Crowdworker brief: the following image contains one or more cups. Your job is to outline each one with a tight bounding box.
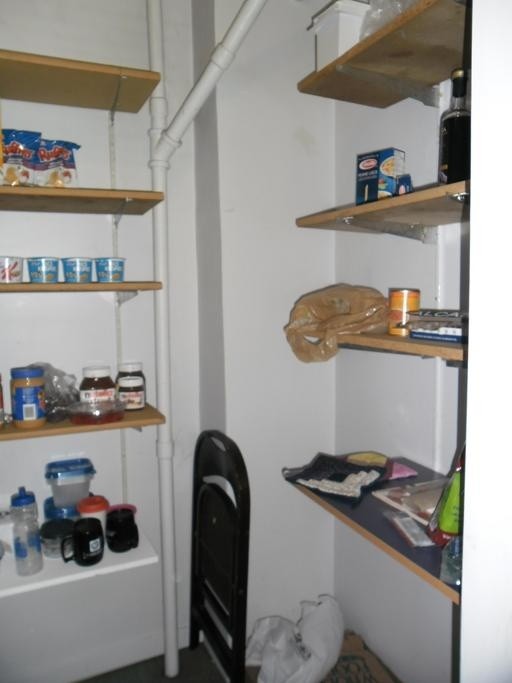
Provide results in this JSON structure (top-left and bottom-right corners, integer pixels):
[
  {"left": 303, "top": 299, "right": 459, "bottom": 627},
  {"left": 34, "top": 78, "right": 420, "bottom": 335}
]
[
  {"left": 61, "top": 517, "right": 104, "bottom": 567},
  {"left": 76, "top": 494, "right": 109, "bottom": 535},
  {"left": 106, "top": 508, "right": 138, "bottom": 553}
]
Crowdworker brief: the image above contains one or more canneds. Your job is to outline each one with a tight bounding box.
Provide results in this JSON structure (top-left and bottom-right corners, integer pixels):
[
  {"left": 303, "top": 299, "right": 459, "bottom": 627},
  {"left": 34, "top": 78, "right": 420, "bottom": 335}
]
[
  {"left": 386, "top": 286, "right": 422, "bottom": 337},
  {"left": 116, "top": 363, "right": 147, "bottom": 413},
  {"left": 10, "top": 366, "right": 48, "bottom": 430},
  {"left": 78, "top": 365, "right": 117, "bottom": 420}
]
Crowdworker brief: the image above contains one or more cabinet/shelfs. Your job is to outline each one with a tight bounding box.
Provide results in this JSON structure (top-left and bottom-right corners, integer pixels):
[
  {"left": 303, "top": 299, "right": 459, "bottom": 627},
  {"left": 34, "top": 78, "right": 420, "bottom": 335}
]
[
  {"left": 282, "top": 0, "right": 462, "bottom": 606},
  {"left": 1, "top": 46, "right": 165, "bottom": 598}
]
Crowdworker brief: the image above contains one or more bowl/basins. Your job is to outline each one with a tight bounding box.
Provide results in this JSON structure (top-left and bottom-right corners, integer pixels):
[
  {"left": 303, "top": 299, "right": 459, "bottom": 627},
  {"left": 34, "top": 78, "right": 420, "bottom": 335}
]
[{"left": 40, "top": 518, "right": 75, "bottom": 560}]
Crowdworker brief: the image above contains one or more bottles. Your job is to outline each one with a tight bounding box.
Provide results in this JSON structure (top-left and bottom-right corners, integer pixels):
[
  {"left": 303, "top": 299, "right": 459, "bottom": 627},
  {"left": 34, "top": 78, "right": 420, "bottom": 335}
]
[
  {"left": 78, "top": 360, "right": 147, "bottom": 413},
  {"left": 439, "top": 65, "right": 471, "bottom": 184},
  {"left": 10, "top": 486, "right": 43, "bottom": 577}
]
